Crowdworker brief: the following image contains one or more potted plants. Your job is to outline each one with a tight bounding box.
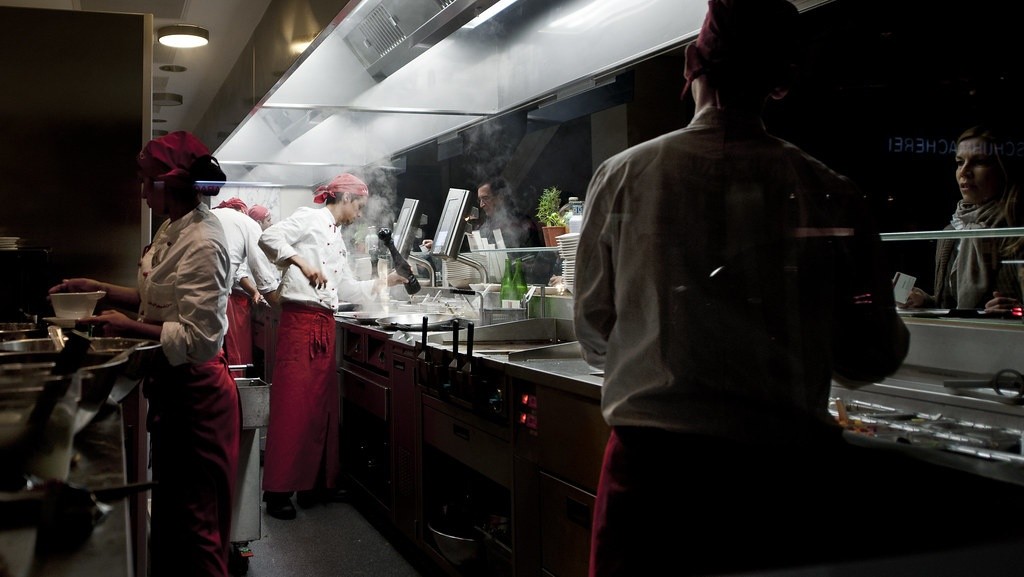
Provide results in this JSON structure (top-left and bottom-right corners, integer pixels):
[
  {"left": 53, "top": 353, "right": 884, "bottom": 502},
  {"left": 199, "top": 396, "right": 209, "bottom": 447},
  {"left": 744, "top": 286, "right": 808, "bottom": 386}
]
[{"left": 535, "top": 186, "right": 567, "bottom": 247}]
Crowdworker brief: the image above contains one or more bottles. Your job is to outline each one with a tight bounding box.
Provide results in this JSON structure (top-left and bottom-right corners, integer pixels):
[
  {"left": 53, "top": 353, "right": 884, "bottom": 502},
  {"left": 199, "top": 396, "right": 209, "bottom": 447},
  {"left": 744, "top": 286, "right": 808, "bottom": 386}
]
[
  {"left": 378, "top": 227, "right": 421, "bottom": 295},
  {"left": 364, "top": 225, "right": 378, "bottom": 252},
  {"left": 559, "top": 196, "right": 583, "bottom": 223},
  {"left": 500, "top": 258, "right": 514, "bottom": 308},
  {"left": 512, "top": 257, "right": 529, "bottom": 309}
]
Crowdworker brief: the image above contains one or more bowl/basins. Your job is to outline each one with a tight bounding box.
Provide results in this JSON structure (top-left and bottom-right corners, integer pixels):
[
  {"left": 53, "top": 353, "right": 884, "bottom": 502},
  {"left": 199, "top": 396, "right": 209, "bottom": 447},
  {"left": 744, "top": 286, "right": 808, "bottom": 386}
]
[
  {"left": 0, "top": 321, "right": 163, "bottom": 490},
  {"left": 336, "top": 303, "right": 457, "bottom": 331},
  {"left": 428, "top": 515, "right": 479, "bottom": 573},
  {"left": 50, "top": 291, "right": 100, "bottom": 318}
]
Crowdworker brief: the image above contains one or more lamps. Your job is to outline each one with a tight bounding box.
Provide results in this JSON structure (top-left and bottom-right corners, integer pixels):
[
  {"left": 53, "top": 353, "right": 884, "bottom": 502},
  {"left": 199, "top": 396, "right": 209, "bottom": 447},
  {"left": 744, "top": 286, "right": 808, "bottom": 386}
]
[
  {"left": 158, "top": 26, "right": 209, "bottom": 48},
  {"left": 153, "top": 93, "right": 182, "bottom": 106}
]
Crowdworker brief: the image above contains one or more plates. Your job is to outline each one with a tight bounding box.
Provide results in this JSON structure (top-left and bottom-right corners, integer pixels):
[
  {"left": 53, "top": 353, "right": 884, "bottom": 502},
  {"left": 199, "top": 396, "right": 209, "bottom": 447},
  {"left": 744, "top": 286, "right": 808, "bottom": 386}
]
[
  {"left": 556, "top": 232, "right": 579, "bottom": 295},
  {"left": 445, "top": 252, "right": 488, "bottom": 288},
  {"left": 44, "top": 317, "right": 94, "bottom": 328},
  {"left": 355, "top": 258, "right": 374, "bottom": 280},
  {"left": 416, "top": 279, "right": 430, "bottom": 285},
  {"left": 0, "top": 237, "right": 21, "bottom": 249}
]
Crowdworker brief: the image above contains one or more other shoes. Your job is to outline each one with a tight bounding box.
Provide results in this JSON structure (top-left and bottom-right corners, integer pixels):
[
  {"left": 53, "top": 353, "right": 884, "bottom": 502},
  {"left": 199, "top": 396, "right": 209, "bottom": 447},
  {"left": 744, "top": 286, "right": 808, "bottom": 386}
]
[
  {"left": 319, "top": 488, "right": 337, "bottom": 496},
  {"left": 267, "top": 495, "right": 296, "bottom": 519}
]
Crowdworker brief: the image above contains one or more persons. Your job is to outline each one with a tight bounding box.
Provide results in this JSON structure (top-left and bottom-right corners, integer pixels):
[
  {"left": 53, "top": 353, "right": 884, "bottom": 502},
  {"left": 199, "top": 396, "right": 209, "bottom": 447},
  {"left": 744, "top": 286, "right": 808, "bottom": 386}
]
[
  {"left": 234, "top": 204, "right": 282, "bottom": 371},
  {"left": 207, "top": 198, "right": 279, "bottom": 380},
  {"left": 573, "top": 1, "right": 909, "bottom": 577},
  {"left": 469, "top": 176, "right": 540, "bottom": 250},
  {"left": 896, "top": 127, "right": 1024, "bottom": 316},
  {"left": 258, "top": 173, "right": 408, "bottom": 521},
  {"left": 48, "top": 129, "right": 244, "bottom": 577}
]
